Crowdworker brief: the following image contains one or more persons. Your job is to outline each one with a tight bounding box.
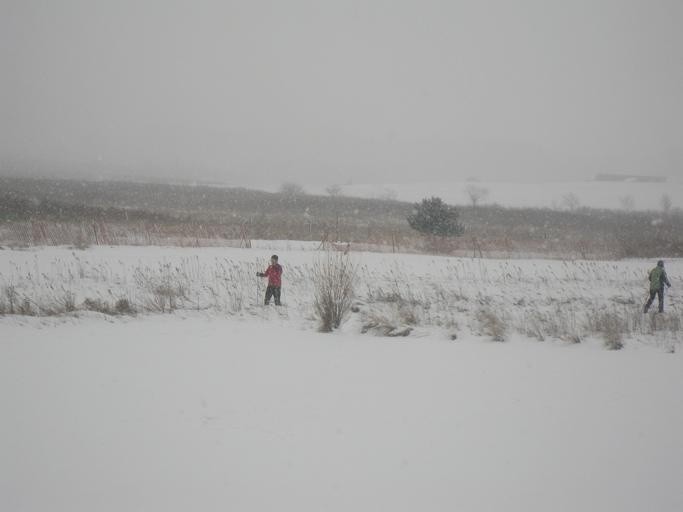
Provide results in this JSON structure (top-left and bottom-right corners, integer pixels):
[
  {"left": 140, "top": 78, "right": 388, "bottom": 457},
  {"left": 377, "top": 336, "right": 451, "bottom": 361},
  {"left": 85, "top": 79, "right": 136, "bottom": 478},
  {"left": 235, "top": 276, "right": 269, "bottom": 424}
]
[
  {"left": 255, "top": 254, "right": 282, "bottom": 305},
  {"left": 643, "top": 260, "right": 671, "bottom": 314}
]
